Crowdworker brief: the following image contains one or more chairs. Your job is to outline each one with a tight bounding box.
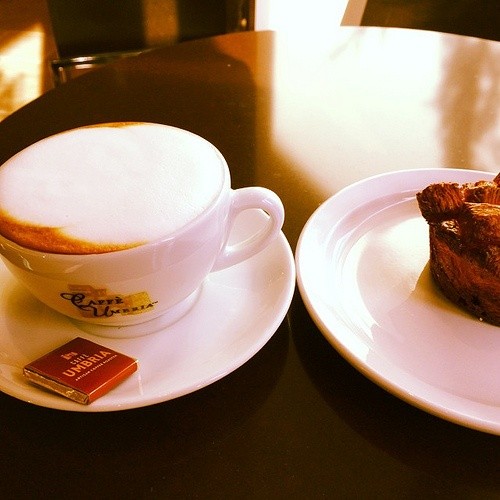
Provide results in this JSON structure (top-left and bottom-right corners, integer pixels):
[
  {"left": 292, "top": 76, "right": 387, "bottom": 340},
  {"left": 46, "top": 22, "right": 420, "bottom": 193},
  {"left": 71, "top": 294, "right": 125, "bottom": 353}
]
[
  {"left": 341, "top": 0, "right": 500, "bottom": 41},
  {"left": 46, "top": 0, "right": 255, "bottom": 88}
]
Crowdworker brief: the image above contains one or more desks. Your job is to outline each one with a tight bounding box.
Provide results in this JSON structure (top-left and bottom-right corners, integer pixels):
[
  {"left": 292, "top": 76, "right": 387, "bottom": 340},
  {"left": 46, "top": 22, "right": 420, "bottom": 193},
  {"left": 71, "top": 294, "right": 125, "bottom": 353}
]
[{"left": 0, "top": 26, "right": 499, "bottom": 500}]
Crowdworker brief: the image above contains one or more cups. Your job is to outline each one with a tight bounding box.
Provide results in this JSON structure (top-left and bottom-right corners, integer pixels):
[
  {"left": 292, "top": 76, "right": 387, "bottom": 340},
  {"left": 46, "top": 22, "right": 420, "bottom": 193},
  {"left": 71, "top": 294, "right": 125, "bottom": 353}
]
[{"left": 0, "top": 119, "right": 285, "bottom": 340}]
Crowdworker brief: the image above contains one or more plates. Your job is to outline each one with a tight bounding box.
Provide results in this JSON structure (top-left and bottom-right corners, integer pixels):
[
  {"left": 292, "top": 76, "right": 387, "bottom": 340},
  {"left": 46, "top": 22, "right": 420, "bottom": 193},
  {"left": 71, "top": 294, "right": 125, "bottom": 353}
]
[
  {"left": 0, "top": 185, "right": 297, "bottom": 414},
  {"left": 295, "top": 166, "right": 500, "bottom": 435}
]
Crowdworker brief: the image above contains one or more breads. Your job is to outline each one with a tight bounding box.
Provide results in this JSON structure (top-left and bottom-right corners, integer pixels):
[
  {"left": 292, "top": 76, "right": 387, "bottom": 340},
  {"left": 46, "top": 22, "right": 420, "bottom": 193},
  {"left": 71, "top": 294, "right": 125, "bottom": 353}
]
[{"left": 416, "top": 172, "right": 500, "bottom": 329}]
